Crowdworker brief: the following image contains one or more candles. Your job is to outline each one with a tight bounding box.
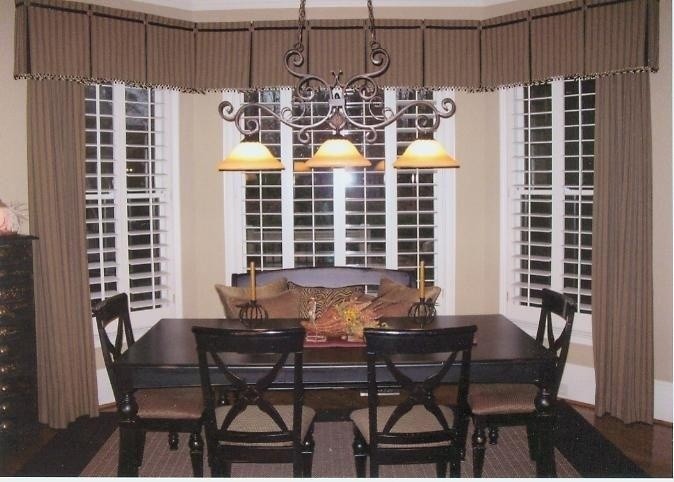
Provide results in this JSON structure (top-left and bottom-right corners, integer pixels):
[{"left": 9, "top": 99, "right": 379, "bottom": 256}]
[
  {"left": 420, "top": 261, "right": 425, "bottom": 298},
  {"left": 250, "top": 261, "right": 255, "bottom": 300}
]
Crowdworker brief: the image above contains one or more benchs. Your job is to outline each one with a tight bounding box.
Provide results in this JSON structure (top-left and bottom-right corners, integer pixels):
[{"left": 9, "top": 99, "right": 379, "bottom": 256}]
[{"left": 231, "top": 267, "right": 414, "bottom": 294}]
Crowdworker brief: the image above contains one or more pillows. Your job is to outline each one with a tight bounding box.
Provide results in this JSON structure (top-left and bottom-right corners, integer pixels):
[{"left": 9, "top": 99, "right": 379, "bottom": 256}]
[
  {"left": 215, "top": 277, "right": 298, "bottom": 319},
  {"left": 372, "top": 277, "right": 441, "bottom": 318},
  {"left": 287, "top": 281, "right": 363, "bottom": 319}
]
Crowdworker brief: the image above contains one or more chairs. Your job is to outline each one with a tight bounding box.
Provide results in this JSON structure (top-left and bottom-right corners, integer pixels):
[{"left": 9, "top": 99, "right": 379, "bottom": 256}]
[
  {"left": 468, "top": 290, "right": 576, "bottom": 476},
  {"left": 345, "top": 323, "right": 478, "bottom": 477},
  {"left": 190, "top": 321, "right": 317, "bottom": 479},
  {"left": 93, "top": 289, "right": 208, "bottom": 477}
]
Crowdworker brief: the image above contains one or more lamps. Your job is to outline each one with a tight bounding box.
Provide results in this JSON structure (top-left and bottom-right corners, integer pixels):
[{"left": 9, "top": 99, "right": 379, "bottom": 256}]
[{"left": 217, "top": 0, "right": 461, "bottom": 171}]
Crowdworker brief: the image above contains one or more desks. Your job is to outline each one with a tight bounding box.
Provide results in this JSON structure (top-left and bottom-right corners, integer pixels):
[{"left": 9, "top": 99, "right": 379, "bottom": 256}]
[{"left": 109, "top": 313, "right": 557, "bottom": 477}]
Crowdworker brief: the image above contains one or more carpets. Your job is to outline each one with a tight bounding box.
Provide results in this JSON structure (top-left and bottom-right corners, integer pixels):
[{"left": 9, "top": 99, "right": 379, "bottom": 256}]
[{"left": 12, "top": 396, "right": 651, "bottom": 478}]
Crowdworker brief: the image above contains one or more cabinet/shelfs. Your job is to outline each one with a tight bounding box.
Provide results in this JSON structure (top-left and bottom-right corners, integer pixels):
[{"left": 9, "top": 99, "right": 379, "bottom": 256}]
[{"left": 0, "top": 235, "right": 41, "bottom": 455}]
